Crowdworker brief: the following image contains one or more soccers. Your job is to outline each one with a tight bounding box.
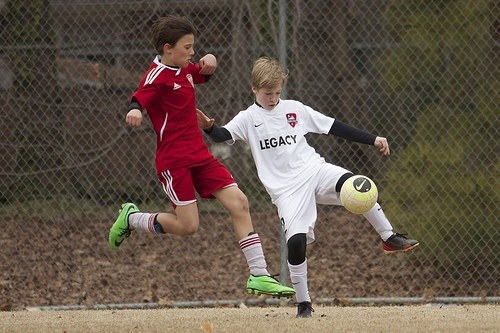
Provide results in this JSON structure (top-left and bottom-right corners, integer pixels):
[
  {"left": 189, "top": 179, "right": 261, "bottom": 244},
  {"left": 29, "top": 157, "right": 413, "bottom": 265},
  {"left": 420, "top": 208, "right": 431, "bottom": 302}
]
[{"left": 340, "top": 175, "right": 378, "bottom": 214}]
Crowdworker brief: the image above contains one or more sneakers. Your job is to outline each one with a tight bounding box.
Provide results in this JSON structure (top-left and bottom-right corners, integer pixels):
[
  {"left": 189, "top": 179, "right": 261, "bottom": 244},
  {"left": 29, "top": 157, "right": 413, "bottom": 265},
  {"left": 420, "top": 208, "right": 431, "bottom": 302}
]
[
  {"left": 294, "top": 301, "right": 314, "bottom": 318},
  {"left": 109, "top": 203, "right": 140, "bottom": 249},
  {"left": 246, "top": 274, "right": 296, "bottom": 299},
  {"left": 379, "top": 232, "right": 419, "bottom": 255}
]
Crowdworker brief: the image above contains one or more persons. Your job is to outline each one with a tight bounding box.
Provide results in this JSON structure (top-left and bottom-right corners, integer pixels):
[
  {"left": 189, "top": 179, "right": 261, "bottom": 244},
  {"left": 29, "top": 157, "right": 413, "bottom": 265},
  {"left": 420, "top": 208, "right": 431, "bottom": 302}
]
[
  {"left": 109, "top": 17, "right": 296, "bottom": 299},
  {"left": 196, "top": 58, "right": 419, "bottom": 318}
]
